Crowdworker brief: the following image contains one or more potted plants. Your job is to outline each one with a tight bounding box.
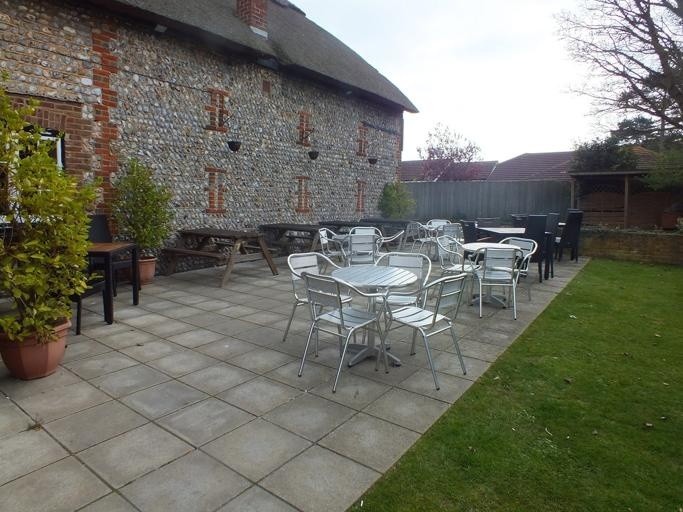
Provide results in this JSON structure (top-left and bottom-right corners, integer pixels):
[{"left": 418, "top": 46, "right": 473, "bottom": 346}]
[
  {"left": 0, "top": 72, "right": 103, "bottom": 380},
  {"left": 106, "top": 157, "right": 175, "bottom": 283}
]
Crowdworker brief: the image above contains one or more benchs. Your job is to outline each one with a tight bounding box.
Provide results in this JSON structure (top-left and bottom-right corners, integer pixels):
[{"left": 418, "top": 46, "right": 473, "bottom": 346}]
[
  {"left": 163, "top": 248, "right": 224, "bottom": 260},
  {"left": 214, "top": 241, "right": 280, "bottom": 256}
]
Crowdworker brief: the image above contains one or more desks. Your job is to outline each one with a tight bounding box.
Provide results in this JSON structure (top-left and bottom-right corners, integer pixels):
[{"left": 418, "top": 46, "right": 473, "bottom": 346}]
[
  {"left": 86, "top": 242, "right": 140, "bottom": 324},
  {"left": 179, "top": 227, "right": 279, "bottom": 289},
  {"left": 259, "top": 222, "right": 337, "bottom": 252}
]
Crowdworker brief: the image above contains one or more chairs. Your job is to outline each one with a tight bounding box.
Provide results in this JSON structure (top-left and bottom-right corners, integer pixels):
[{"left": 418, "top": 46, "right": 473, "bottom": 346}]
[{"left": 73, "top": 279, "right": 109, "bottom": 335}]
